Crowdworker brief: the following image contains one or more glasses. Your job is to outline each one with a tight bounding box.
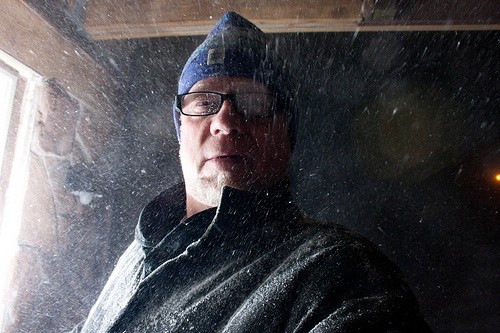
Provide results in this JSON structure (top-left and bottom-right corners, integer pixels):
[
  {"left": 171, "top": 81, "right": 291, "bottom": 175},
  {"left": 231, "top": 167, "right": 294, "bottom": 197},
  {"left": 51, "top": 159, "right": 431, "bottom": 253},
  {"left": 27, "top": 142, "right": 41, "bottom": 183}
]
[{"left": 176, "top": 91, "right": 280, "bottom": 117}]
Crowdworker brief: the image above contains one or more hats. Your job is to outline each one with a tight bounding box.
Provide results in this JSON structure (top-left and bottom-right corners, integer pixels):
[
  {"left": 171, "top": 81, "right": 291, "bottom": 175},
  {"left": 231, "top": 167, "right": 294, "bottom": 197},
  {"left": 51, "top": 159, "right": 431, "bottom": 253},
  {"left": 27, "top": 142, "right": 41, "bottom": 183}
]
[{"left": 172, "top": 10, "right": 286, "bottom": 144}]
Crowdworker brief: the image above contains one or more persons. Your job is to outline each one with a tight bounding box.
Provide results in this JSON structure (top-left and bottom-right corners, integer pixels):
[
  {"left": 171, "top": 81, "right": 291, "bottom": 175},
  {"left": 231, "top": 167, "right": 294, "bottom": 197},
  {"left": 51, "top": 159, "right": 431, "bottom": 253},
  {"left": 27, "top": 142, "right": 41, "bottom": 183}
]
[{"left": 68, "top": 11, "right": 433, "bottom": 333}]
